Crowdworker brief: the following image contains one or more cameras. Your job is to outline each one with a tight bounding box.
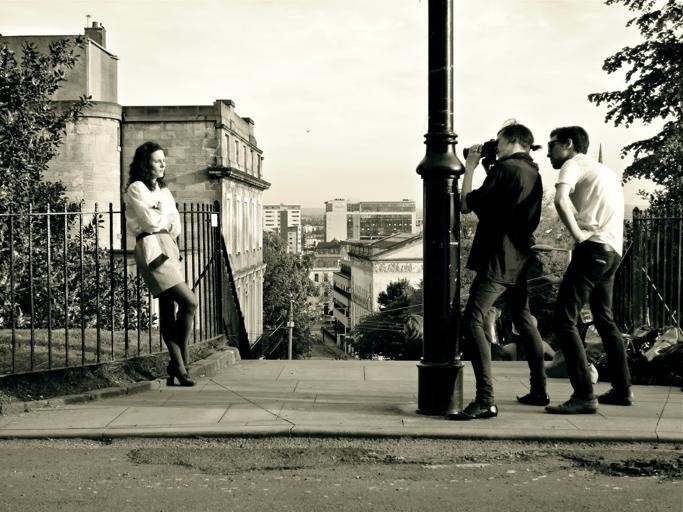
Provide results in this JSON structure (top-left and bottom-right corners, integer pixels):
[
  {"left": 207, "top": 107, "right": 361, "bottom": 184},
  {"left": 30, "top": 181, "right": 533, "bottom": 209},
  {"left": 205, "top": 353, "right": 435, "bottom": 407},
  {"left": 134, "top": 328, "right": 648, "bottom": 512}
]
[{"left": 463, "top": 138, "right": 498, "bottom": 174}]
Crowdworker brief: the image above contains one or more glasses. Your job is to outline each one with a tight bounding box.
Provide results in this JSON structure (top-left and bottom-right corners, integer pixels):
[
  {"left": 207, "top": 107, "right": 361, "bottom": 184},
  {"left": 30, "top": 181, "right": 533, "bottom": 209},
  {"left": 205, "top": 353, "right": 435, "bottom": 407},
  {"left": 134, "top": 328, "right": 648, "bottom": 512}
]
[{"left": 547, "top": 139, "right": 559, "bottom": 148}]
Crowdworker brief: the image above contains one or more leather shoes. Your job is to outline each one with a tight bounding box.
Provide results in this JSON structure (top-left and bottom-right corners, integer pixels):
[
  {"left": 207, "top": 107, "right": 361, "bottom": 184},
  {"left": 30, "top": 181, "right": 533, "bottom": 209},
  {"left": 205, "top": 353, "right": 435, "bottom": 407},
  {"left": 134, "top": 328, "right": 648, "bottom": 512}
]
[
  {"left": 545, "top": 396, "right": 600, "bottom": 413},
  {"left": 597, "top": 388, "right": 632, "bottom": 405},
  {"left": 518, "top": 393, "right": 550, "bottom": 406},
  {"left": 449, "top": 402, "right": 498, "bottom": 419}
]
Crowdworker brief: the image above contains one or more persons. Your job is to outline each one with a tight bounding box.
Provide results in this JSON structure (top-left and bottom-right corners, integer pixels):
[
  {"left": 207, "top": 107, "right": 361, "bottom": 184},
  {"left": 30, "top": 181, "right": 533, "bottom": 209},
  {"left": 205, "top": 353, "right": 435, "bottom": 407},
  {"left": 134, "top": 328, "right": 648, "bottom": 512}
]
[
  {"left": 127, "top": 141, "right": 198, "bottom": 386},
  {"left": 446, "top": 122, "right": 551, "bottom": 421},
  {"left": 544, "top": 127, "right": 632, "bottom": 413}
]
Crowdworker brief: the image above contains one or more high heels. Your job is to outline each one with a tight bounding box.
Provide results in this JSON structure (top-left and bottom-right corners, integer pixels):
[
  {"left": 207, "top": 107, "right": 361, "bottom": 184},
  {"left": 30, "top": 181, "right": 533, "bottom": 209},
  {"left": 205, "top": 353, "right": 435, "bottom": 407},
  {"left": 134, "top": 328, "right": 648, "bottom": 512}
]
[{"left": 167, "top": 360, "right": 196, "bottom": 386}]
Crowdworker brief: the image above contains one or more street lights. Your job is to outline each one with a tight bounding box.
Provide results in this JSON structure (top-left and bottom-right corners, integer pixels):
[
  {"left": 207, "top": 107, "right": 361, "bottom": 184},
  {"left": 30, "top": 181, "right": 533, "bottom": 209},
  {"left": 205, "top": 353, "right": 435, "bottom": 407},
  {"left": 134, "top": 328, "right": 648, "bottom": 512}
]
[
  {"left": 336, "top": 307, "right": 347, "bottom": 356},
  {"left": 287, "top": 299, "right": 296, "bottom": 360}
]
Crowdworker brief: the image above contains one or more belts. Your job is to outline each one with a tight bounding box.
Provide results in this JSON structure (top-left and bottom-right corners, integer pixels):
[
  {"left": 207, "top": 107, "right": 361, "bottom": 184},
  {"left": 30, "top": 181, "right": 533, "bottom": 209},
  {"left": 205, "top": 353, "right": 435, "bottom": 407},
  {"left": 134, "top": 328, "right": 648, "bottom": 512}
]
[{"left": 581, "top": 242, "right": 614, "bottom": 252}]
